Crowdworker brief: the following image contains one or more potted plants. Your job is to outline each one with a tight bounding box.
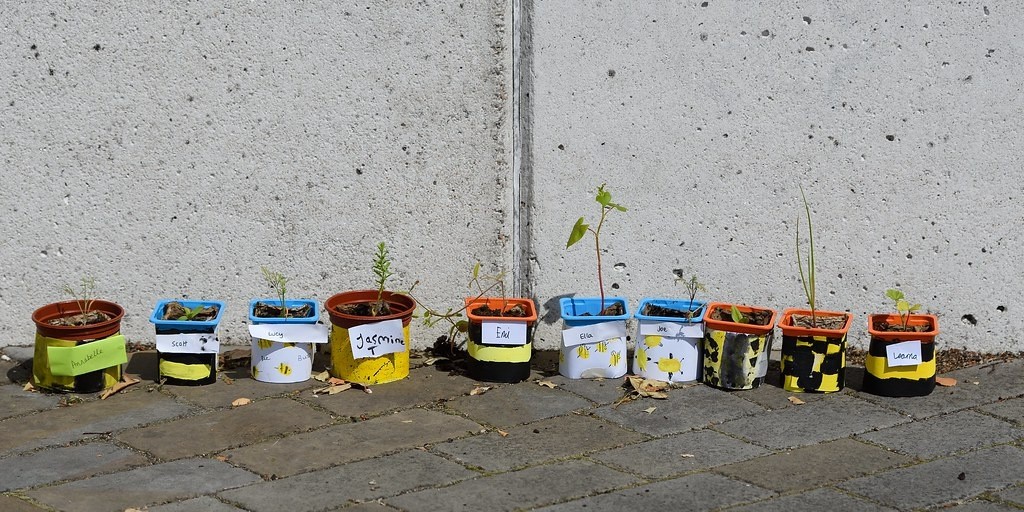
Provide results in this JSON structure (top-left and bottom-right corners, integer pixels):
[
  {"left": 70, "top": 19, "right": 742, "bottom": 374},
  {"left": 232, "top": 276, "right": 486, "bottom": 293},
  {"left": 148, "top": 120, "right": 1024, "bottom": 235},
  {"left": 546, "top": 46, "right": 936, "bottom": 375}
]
[
  {"left": 247, "top": 266, "right": 329, "bottom": 384},
  {"left": 861, "top": 289, "right": 938, "bottom": 399},
  {"left": 632, "top": 267, "right": 708, "bottom": 382},
  {"left": 703, "top": 304, "right": 778, "bottom": 391},
  {"left": 390, "top": 260, "right": 538, "bottom": 383},
  {"left": 558, "top": 183, "right": 631, "bottom": 379},
  {"left": 33, "top": 277, "right": 128, "bottom": 393},
  {"left": 324, "top": 242, "right": 416, "bottom": 387},
  {"left": 776, "top": 183, "right": 854, "bottom": 393},
  {"left": 149, "top": 300, "right": 224, "bottom": 386}
]
